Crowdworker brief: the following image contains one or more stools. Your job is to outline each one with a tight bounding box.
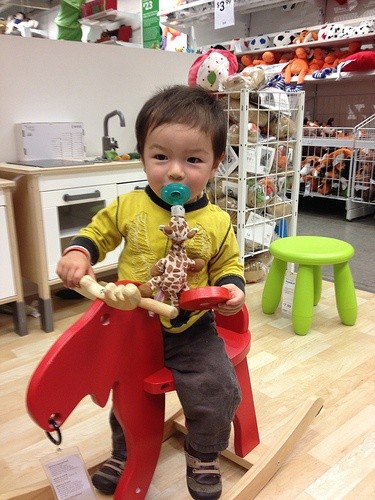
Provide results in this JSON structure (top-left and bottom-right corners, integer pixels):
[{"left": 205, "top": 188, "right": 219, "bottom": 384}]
[{"left": 261, "top": 235, "right": 357, "bottom": 336}]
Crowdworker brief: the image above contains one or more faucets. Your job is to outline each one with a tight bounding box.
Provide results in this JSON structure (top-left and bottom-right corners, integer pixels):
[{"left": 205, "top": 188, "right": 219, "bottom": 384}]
[{"left": 101, "top": 109, "right": 126, "bottom": 159}]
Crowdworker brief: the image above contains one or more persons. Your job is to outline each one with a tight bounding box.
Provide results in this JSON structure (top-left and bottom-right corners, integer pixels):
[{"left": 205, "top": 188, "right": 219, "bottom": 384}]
[{"left": 55, "top": 84, "right": 247, "bottom": 500}]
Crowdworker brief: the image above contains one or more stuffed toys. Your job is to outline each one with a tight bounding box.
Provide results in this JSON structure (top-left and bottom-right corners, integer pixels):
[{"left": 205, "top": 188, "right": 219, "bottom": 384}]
[
  {"left": 301, "top": 118, "right": 375, "bottom": 201},
  {"left": 187, "top": 48, "right": 291, "bottom": 283},
  {"left": 139, "top": 205, "right": 205, "bottom": 312},
  {"left": 241, "top": 40, "right": 375, "bottom": 93}
]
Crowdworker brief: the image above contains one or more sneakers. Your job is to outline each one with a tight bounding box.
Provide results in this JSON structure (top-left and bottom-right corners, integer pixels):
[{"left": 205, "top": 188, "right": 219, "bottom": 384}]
[
  {"left": 91, "top": 454, "right": 126, "bottom": 490},
  {"left": 184, "top": 436, "right": 222, "bottom": 500}
]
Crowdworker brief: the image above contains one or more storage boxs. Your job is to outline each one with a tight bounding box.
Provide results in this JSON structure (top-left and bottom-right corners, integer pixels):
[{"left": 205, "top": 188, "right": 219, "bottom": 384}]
[{"left": 14, "top": 121, "right": 87, "bottom": 163}]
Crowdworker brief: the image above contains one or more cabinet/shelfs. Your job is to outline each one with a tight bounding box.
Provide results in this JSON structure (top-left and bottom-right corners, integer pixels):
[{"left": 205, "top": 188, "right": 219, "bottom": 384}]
[
  {"left": 0, "top": 169, "right": 151, "bottom": 334},
  {"left": 77, "top": 8, "right": 143, "bottom": 49},
  {"left": 156, "top": 0, "right": 375, "bottom": 150}
]
